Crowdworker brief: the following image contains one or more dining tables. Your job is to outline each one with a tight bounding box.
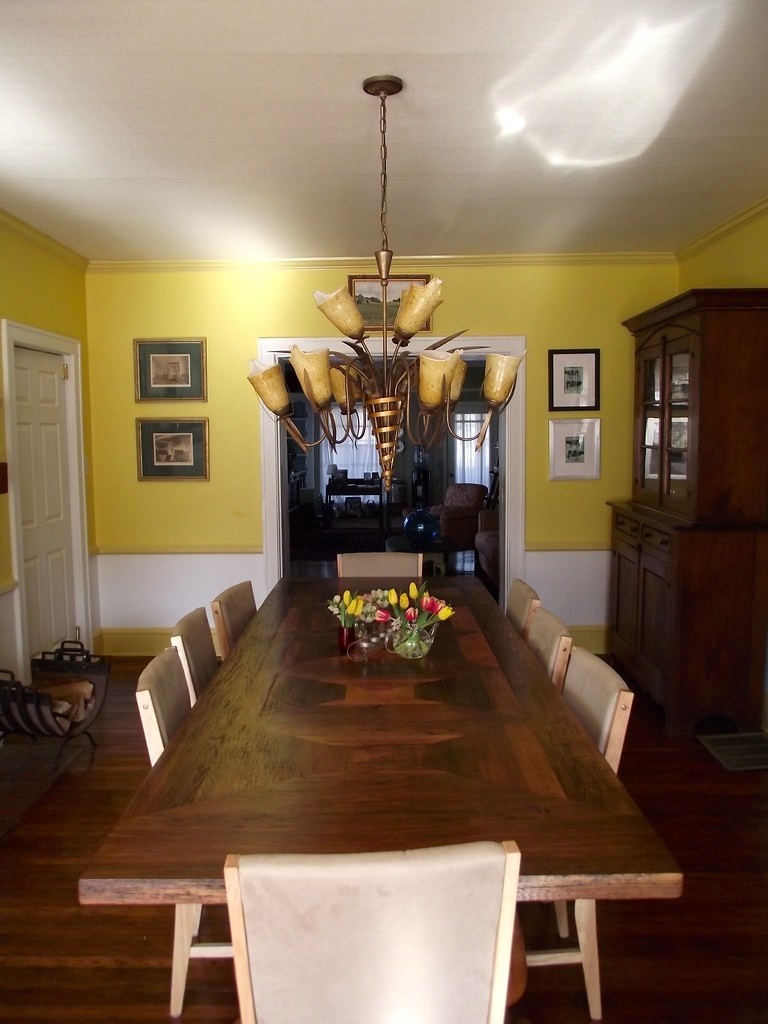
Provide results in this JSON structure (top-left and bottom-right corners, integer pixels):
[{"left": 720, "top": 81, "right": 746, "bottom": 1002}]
[{"left": 73, "top": 572, "right": 685, "bottom": 1024}]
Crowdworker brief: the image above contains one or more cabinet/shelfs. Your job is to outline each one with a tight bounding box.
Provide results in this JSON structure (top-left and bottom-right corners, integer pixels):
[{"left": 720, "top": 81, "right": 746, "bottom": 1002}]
[
  {"left": 603, "top": 501, "right": 687, "bottom": 734},
  {"left": 619, "top": 292, "right": 703, "bottom": 529}
]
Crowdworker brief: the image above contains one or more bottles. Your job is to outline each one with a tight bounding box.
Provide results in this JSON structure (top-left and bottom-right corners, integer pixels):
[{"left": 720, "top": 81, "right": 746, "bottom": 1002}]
[{"left": 413, "top": 444, "right": 429, "bottom": 467}]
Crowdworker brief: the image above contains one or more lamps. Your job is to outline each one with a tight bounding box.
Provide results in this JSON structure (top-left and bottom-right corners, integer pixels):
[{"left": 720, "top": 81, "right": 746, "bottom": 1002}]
[
  {"left": 327, "top": 464, "right": 339, "bottom": 489},
  {"left": 135, "top": 416, "right": 212, "bottom": 484},
  {"left": 248, "top": 71, "right": 527, "bottom": 493},
  {"left": 548, "top": 418, "right": 601, "bottom": 482},
  {"left": 547, "top": 347, "right": 601, "bottom": 413},
  {"left": 132, "top": 336, "right": 210, "bottom": 405}
]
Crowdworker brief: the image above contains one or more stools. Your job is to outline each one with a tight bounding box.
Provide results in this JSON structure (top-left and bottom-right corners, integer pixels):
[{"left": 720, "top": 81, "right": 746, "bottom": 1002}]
[{"left": 1, "top": 639, "right": 112, "bottom": 775}]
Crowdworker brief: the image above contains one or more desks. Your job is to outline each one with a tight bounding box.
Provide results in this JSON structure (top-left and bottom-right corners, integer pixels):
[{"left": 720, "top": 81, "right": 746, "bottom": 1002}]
[{"left": 324, "top": 478, "right": 384, "bottom": 534}]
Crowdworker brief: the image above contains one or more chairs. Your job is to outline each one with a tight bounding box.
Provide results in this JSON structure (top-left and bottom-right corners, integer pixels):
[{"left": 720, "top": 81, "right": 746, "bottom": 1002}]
[
  {"left": 524, "top": 603, "right": 573, "bottom": 700},
  {"left": 134, "top": 646, "right": 193, "bottom": 770},
  {"left": 508, "top": 576, "right": 541, "bottom": 641},
  {"left": 211, "top": 580, "right": 261, "bottom": 664},
  {"left": 475, "top": 509, "right": 501, "bottom": 588},
  {"left": 522, "top": 646, "right": 636, "bottom": 1021},
  {"left": 168, "top": 608, "right": 220, "bottom": 709},
  {"left": 220, "top": 839, "right": 524, "bottom": 1024},
  {"left": 401, "top": 482, "right": 488, "bottom": 553},
  {"left": 336, "top": 552, "right": 423, "bottom": 578}
]
